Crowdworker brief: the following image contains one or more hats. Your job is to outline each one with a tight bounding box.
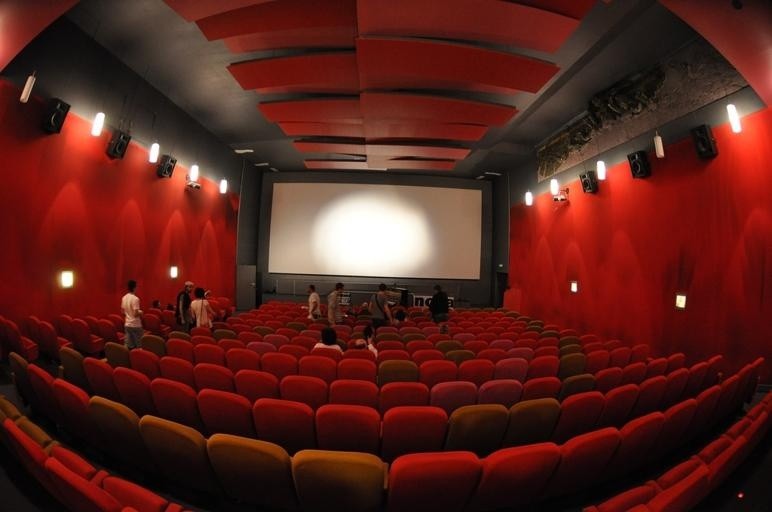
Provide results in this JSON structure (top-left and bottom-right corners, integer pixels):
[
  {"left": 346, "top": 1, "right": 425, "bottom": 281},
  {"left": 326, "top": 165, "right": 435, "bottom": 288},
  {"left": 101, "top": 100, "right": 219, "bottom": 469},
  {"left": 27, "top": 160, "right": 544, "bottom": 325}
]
[{"left": 185, "top": 281, "right": 194, "bottom": 285}]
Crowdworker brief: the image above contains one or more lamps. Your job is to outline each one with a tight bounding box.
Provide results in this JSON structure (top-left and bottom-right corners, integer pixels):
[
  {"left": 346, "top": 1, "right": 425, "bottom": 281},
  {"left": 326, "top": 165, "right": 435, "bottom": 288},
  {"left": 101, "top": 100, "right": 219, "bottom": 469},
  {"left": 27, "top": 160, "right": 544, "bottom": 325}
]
[
  {"left": 721, "top": 86, "right": 741, "bottom": 132},
  {"left": 596, "top": 133, "right": 605, "bottom": 180},
  {"left": 219, "top": 174, "right": 228, "bottom": 194},
  {"left": 148, "top": 138, "right": 159, "bottom": 163},
  {"left": 189, "top": 164, "right": 199, "bottom": 182},
  {"left": 550, "top": 161, "right": 559, "bottom": 197},
  {"left": 525, "top": 185, "right": 533, "bottom": 207},
  {"left": 92, "top": 108, "right": 105, "bottom": 136},
  {"left": 651, "top": 108, "right": 665, "bottom": 158},
  {"left": 19, "top": 70, "right": 37, "bottom": 103}
]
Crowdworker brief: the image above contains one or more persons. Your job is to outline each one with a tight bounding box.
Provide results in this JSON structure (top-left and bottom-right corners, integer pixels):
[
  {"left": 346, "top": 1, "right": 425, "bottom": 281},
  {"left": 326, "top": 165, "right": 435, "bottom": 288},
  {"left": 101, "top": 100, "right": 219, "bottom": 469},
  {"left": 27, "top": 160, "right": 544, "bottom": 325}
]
[
  {"left": 166, "top": 303, "right": 174, "bottom": 311},
  {"left": 429, "top": 284, "right": 449, "bottom": 323},
  {"left": 306, "top": 284, "right": 322, "bottom": 320},
  {"left": 312, "top": 327, "right": 344, "bottom": 354},
  {"left": 350, "top": 333, "right": 378, "bottom": 358},
  {"left": 359, "top": 302, "right": 371, "bottom": 315},
  {"left": 327, "top": 283, "right": 345, "bottom": 325},
  {"left": 368, "top": 284, "right": 392, "bottom": 328},
  {"left": 152, "top": 300, "right": 161, "bottom": 308},
  {"left": 176, "top": 281, "right": 194, "bottom": 332},
  {"left": 121, "top": 280, "right": 144, "bottom": 350},
  {"left": 189, "top": 288, "right": 216, "bottom": 329},
  {"left": 393, "top": 310, "right": 408, "bottom": 322}
]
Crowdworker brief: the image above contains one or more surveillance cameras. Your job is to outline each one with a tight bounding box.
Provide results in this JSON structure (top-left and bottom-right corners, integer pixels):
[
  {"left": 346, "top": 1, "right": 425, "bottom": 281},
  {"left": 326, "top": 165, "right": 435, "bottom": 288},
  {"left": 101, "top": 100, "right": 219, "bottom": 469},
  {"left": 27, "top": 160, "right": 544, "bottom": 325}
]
[
  {"left": 186, "top": 182, "right": 193, "bottom": 188},
  {"left": 553, "top": 196, "right": 558, "bottom": 201},
  {"left": 193, "top": 183, "right": 201, "bottom": 190},
  {"left": 559, "top": 196, "right": 565, "bottom": 201}
]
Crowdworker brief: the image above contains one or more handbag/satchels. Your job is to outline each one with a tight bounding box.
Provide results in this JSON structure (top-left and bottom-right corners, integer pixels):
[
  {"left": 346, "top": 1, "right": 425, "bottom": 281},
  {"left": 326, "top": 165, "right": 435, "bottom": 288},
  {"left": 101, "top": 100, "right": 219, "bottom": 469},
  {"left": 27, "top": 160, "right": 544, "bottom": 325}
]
[
  {"left": 384, "top": 310, "right": 394, "bottom": 320},
  {"left": 201, "top": 323, "right": 209, "bottom": 328}
]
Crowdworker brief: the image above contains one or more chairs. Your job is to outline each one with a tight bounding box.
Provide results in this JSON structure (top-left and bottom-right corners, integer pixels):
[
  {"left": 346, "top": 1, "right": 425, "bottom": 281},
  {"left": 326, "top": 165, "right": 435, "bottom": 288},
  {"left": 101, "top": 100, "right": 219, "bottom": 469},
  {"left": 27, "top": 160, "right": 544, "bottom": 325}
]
[{"left": 0, "top": 297, "right": 772, "bottom": 512}]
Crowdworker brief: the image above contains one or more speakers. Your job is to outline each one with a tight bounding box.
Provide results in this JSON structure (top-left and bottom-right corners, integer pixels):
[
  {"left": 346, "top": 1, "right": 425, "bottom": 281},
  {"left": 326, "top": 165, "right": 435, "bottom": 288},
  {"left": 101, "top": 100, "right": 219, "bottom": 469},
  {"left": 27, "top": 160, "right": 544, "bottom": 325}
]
[
  {"left": 40, "top": 98, "right": 71, "bottom": 134},
  {"left": 579, "top": 171, "right": 597, "bottom": 193},
  {"left": 627, "top": 150, "right": 650, "bottom": 177},
  {"left": 157, "top": 154, "right": 177, "bottom": 179},
  {"left": 106, "top": 130, "right": 132, "bottom": 160},
  {"left": 690, "top": 124, "right": 718, "bottom": 157}
]
